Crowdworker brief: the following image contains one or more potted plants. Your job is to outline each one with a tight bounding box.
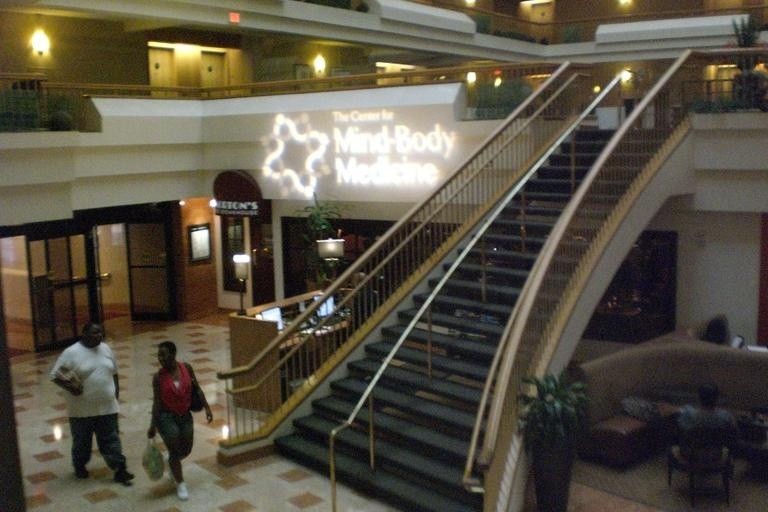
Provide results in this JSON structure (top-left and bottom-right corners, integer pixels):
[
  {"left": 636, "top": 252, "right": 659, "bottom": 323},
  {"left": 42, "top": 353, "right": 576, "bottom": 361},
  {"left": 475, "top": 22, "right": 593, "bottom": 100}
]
[
  {"left": 721, "top": 11, "right": 767, "bottom": 103},
  {"left": 520, "top": 368, "right": 590, "bottom": 511}
]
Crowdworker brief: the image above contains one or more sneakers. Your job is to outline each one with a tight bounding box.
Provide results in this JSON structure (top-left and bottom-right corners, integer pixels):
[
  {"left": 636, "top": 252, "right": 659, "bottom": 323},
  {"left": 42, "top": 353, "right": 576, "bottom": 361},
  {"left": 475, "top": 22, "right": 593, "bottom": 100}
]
[
  {"left": 73, "top": 465, "right": 90, "bottom": 479},
  {"left": 113, "top": 468, "right": 136, "bottom": 482},
  {"left": 164, "top": 453, "right": 175, "bottom": 481},
  {"left": 175, "top": 480, "right": 190, "bottom": 502}
]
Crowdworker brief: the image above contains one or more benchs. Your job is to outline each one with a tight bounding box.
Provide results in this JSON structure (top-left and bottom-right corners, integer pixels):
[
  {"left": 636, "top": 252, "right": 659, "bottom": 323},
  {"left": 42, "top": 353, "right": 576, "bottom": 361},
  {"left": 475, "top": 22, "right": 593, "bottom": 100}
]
[{"left": 576, "top": 339, "right": 768, "bottom": 470}]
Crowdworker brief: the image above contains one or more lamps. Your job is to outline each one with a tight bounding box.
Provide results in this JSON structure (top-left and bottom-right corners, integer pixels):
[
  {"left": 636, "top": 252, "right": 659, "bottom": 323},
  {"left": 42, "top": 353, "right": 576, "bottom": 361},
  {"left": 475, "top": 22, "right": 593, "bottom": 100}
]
[
  {"left": 232, "top": 254, "right": 250, "bottom": 315},
  {"left": 313, "top": 236, "right": 346, "bottom": 266}
]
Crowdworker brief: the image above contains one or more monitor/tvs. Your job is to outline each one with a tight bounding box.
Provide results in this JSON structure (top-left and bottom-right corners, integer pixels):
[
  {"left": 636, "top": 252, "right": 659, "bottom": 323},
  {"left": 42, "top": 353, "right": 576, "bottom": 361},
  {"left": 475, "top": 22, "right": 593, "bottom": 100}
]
[
  {"left": 313, "top": 294, "right": 335, "bottom": 317},
  {"left": 731, "top": 335, "right": 745, "bottom": 349},
  {"left": 261, "top": 306, "right": 283, "bottom": 331}
]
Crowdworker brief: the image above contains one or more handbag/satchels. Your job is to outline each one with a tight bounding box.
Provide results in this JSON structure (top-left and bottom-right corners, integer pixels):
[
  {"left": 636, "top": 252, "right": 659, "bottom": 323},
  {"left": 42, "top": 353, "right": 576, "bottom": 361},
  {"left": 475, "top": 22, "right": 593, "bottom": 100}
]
[{"left": 183, "top": 361, "right": 205, "bottom": 413}]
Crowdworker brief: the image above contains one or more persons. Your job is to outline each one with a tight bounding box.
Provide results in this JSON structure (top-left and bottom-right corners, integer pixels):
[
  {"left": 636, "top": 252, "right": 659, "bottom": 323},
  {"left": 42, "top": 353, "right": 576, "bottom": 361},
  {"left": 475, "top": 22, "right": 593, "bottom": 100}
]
[
  {"left": 49, "top": 322, "right": 136, "bottom": 483},
  {"left": 703, "top": 315, "right": 732, "bottom": 346},
  {"left": 145, "top": 340, "right": 214, "bottom": 503},
  {"left": 670, "top": 380, "right": 742, "bottom": 450}
]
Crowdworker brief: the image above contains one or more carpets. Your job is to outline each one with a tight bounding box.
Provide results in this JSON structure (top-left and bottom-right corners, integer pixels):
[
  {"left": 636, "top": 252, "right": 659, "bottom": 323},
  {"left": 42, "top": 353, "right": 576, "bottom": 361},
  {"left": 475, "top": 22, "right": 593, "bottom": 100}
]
[
  {"left": 66, "top": 306, "right": 130, "bottom": 324},
  {"left": 562, "top": 444, "right": 767, "bottom": 511},
  {"left": 8, "top": 344, "right": 30, "bottom": 359}
]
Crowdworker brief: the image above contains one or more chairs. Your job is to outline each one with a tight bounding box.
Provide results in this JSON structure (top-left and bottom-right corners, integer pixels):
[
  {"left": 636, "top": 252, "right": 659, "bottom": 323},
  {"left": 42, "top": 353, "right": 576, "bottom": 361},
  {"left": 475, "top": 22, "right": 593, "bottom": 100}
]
[{"left": 667, "top": 431, "right": 735, "bottom": 508}]
